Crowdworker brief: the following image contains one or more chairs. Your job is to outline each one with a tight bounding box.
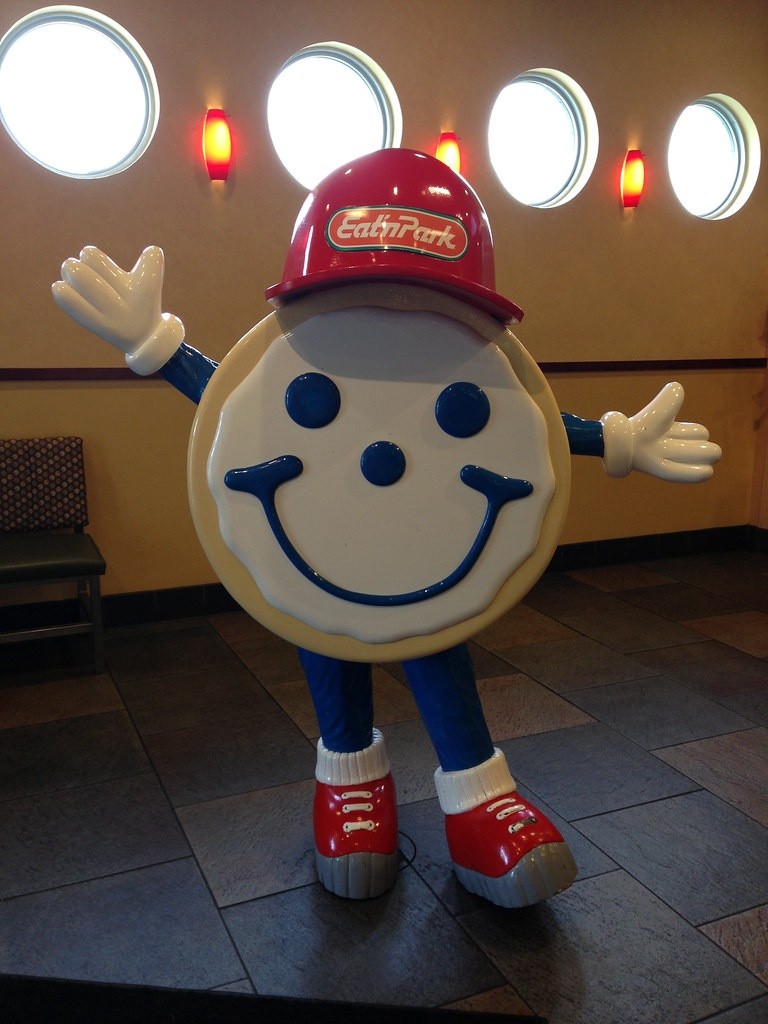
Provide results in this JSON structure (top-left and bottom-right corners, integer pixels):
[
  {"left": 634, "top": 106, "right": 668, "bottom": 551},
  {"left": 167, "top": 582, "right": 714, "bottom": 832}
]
[{"left": 0, "top": 434, "right": 105, "bottom": 674}]
